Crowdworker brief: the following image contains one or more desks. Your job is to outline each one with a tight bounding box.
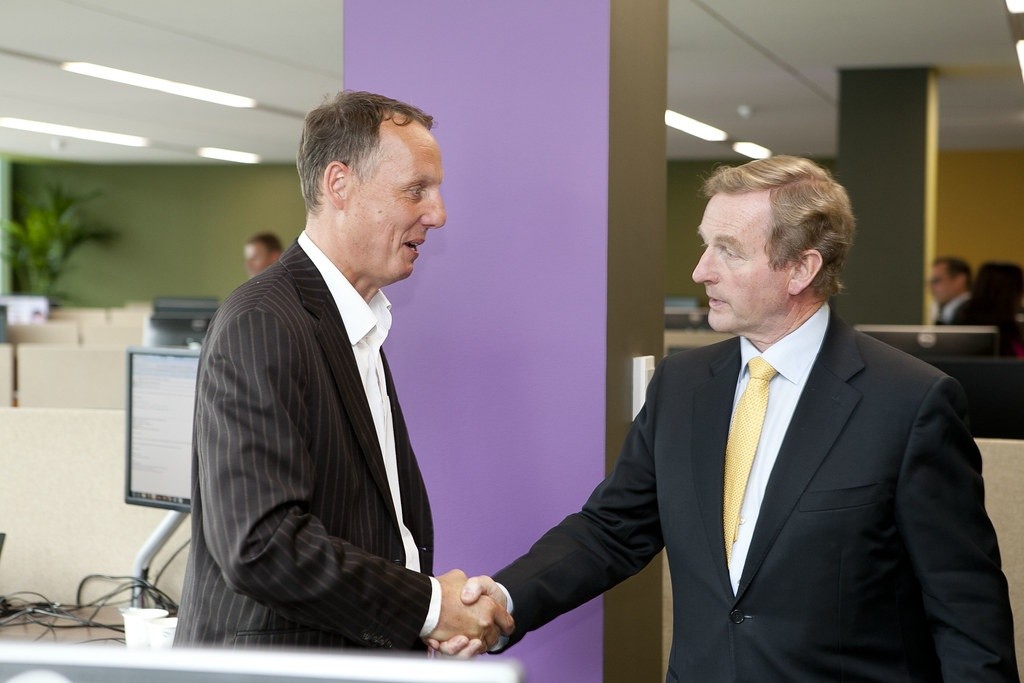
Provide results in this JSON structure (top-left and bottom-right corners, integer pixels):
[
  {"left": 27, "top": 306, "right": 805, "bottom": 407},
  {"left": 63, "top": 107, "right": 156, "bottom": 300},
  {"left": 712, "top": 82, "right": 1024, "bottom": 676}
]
[{"left": 0, "top": 605, "right": 125, "bottom": 647}]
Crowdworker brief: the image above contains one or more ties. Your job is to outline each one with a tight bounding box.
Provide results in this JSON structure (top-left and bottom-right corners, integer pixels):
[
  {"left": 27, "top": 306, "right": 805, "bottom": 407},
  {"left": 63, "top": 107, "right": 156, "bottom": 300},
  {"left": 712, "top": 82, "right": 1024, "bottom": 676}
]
[
  {"left": 930, "top": 274, "right": 952, "bottom": 286},
  {"left": 723, "top": 356, "right": 777, "bottom": 561}
]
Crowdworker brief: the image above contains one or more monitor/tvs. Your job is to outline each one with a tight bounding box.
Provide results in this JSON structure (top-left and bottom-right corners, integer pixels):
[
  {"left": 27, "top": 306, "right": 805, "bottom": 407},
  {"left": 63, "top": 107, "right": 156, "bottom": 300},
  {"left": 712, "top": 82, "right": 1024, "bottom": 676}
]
[
  {"left": 661, "top": 295, "right": 716, "bottom": 331},
  {"left": 851, "top": 325, "right": 1024, "bottom": 439},
  {"left": 123, "top": 295, "right": 223, "bottom": 607},
  {"left": 0, "top": 292, "right": 52, "bottom": 345}
]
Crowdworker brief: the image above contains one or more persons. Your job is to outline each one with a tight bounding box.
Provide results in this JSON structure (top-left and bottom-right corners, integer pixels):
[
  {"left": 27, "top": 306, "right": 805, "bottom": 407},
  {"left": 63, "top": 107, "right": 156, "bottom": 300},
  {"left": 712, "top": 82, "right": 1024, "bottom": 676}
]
[
  {"left": 930, "top": 254, "right": 975, "bottom": 325},
  {"left": 243, "top": 233, "right": 282, "bottom": 279},
  {"left": 949, "top": 261, "right": 1024, "bottom": 358},
  {"left": 170, "top": 91, "right": 515, "bottom": 659},
  {"left": 421, "top": 154, "right": 1021, "bottom": 683}
]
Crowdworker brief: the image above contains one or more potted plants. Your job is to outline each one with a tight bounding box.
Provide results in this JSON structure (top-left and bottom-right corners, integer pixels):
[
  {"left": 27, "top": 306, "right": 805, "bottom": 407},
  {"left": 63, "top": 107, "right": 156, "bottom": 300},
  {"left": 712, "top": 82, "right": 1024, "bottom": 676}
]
[{"left": 0, "top": 177, "right": 123, "bottom": 309}]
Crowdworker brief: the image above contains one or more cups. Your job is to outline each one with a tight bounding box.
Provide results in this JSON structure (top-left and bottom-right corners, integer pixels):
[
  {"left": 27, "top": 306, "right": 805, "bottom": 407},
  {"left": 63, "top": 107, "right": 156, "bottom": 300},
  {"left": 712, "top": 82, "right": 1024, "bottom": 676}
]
[
  {"left": 119, "top": 608, "right": 169, "bottom": 647},
  {"left": 146, "top": 617, "right": 178, "bottom": 648}
]
